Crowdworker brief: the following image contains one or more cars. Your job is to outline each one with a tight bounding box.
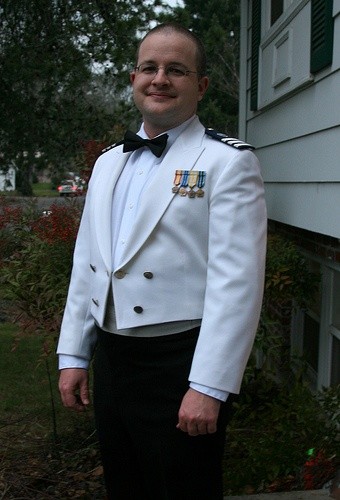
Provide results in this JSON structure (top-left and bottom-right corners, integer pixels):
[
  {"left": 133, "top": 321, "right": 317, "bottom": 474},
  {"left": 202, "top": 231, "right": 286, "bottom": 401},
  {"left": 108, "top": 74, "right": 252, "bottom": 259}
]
[{"left": 58, "top": 180, "right": 84, "bottom": 197}]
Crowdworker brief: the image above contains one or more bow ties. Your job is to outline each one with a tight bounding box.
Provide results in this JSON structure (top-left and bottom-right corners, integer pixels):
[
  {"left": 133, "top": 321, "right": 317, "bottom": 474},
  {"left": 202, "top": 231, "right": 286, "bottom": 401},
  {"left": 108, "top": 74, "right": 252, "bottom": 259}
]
[{"left": 122, "top": 130, "right": 169, "bottom": 157}]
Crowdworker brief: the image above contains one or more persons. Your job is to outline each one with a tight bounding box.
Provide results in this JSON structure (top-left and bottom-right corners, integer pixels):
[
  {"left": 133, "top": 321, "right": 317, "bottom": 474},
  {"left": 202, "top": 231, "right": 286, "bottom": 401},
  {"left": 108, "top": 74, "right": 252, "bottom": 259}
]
[{"left": 54, "top": 20, "right": 270, "bottom": 500}]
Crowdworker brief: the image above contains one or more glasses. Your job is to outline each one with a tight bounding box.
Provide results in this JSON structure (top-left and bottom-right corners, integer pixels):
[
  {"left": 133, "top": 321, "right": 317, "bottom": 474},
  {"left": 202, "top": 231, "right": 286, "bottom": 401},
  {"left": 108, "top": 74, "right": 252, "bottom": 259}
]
[{"left": 133, "top": 63, "right": 204, "bottom": 80}]
